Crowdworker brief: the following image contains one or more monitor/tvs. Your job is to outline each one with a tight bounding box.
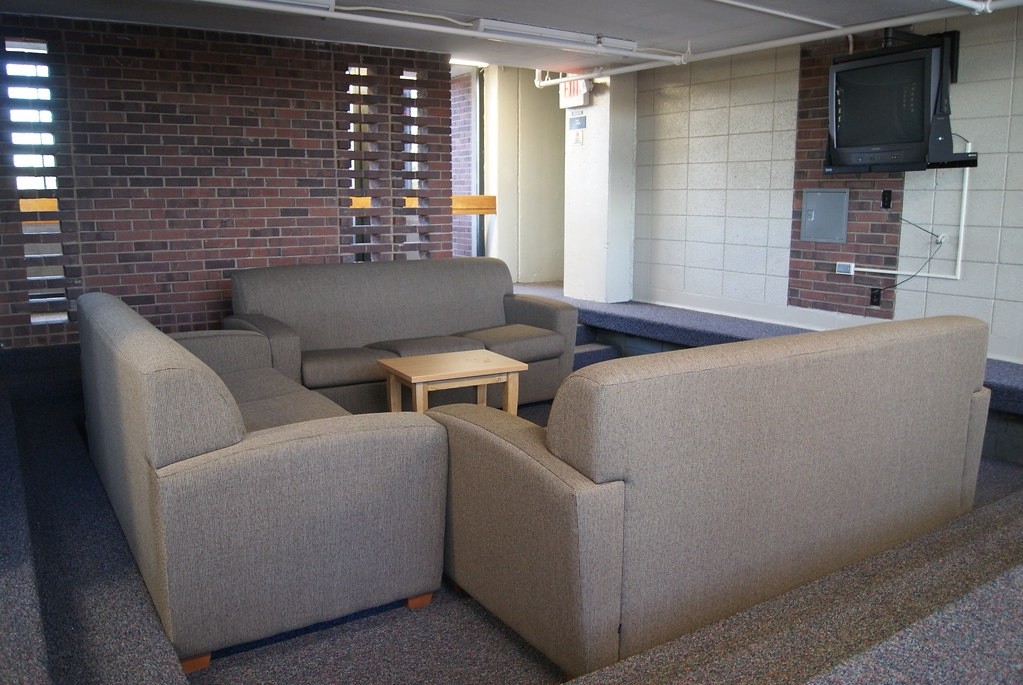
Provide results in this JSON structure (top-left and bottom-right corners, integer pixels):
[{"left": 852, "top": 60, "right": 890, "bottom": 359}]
[{"left": 827, "top": 48, "right": 941, "bottom": 165}]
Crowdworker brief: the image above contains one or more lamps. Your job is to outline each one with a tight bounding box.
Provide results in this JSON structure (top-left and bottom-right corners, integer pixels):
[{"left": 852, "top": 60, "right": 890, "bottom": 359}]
[
  {"left": 220, "top": 0, "right": 335, "bottom": 22},
  {"left": 467, "top": 19, "right": 637, "bottom": 59}
]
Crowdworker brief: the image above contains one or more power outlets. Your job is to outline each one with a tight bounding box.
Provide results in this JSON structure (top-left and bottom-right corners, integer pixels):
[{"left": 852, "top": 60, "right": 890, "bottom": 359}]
[{"left": 870, "top": 288, "right": 881, "bottom": 306}]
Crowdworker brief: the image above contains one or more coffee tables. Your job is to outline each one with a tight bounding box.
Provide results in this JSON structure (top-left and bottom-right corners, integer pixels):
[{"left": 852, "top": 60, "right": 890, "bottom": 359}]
[{"left": 376, "top": 349, "right": 529, "bottom": 416}]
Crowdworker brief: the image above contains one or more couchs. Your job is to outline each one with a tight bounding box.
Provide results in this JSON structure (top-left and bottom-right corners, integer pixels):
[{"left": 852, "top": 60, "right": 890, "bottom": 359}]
[
  {"left": 77, "top": 292, "right": 449, "bottom": 674},
  {"left": 222, "top": 255, "right": 579, "bottom": 416},
  {"left": 422, "top": 314, "right": 991, "bottom": 677}
]
